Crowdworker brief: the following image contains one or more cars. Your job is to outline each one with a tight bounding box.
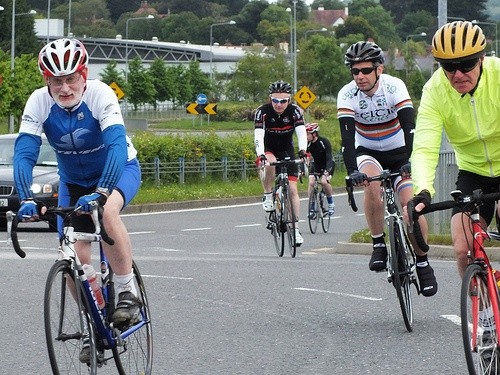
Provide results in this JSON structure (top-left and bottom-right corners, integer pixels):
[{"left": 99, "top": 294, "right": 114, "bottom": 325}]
[{"left": 0, "top": 132, "right": 61, "bottom": 231}]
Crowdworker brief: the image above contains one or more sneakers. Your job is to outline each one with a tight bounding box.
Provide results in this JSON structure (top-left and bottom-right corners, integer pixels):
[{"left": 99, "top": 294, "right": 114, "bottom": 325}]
[
  {"left": 369, "top": 243, "right": 388, "bottom": 271},
  {"left": 328, "top": 201, "right": 335, "bottom": 215},
  {"left": 79, "top": 331, "right": 102, "bottom": 362},
  {"left": 416, "top": 261, "right": 437, "bottom": 297},
  {"left": 307, "top": 209, "right": 314, "bottom": 218},
  {"left": 289, "top": 228, "right": 304, "bottom": 247},
  {"left": 112, "top": 291, "right": 143, "bottom": 327},
  {"left": 262, "top": 192, "right": 274, "bottom": 212}
]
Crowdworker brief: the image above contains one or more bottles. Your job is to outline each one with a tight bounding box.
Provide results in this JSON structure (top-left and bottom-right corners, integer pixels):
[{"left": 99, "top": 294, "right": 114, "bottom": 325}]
[
  {"left": 492, "top": 270, "right": 500, "bottom": 295},
  {"left": 81, "top": 264, "right": 106, "bottom": 311}
]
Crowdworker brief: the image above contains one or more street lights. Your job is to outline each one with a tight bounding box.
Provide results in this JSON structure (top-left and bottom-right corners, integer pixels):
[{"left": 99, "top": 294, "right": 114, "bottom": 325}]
[
  {"left": 210, "top": 21, "right": 236, "bottom": 96},
  {"left": 286, "top": 8, "right": 294, "bottom": 65},
  {"left": 472, "top": 20, "right": 498, "bottom": 57},
  {"left": 406, "top": 32, "right": 427, "bottom": 56},
  {"left": 8, "top": 10, "right": 36, "bottom": 132},
  {"left": 125, "top": 15, "right": 155, "bottom": 117},
  {"left": 305, "top": 28, "right": 327, "bottom": 42}
]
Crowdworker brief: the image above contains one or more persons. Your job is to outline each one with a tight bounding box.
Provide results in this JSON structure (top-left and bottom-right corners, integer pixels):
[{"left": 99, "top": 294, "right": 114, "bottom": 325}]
[
  {"left": 12, "top": 38, "right": 141, "bottom": 364},
  {"left": 337, "top": 40, "right": 439, "bottom": 297},
  {"left": 403, "top": 21, "right": 499, "bottom": 352},
  {"left": 254, "top": 79, "right": 335, "bottom": 246}
]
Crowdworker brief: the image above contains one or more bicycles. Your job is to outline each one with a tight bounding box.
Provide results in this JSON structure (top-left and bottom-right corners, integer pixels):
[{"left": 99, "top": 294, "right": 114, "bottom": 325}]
[
  {"left": 10, "top": 206, "right": 154, "bottom": 375},
  {"left": 343, "top": 173, "right": 422, "bottom": 332},
  {"left": 299, "top": 168, "right": 330, "bottom": 234},
  {"left": 260, "top": 150, "right": 311, "bottom": 258},
  {"left": 407, "top": 188, "right": 500, "bottom": 375}
]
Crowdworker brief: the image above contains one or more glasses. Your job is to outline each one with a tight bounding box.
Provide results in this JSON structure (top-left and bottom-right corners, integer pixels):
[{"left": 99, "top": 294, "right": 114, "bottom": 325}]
[
  {"left": 440, "top": 57, "right": 479, "bottom": 73},
  {"left": 47, "top": 74, "right": 82, "bottom": 87},
  {"left": 349, "top": 67, "right": 376, "bottom": 75},
  {"left": 271, "top": 96, "right": 290, "bottom": 103}
]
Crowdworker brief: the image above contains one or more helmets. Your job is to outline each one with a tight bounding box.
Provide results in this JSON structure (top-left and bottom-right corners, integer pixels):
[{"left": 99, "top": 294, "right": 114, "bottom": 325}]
[
  {"left": 37, "top": 37, "right": 89, "bottom": 79},
  {"left": 268, "top": 80, "right": 293, "bottom": 94},
  {"left": 344, "top": 40, "right": 385, "bottom": 65},
  {"left": 431, "top": 21, "right": 487, "bottom": 63},
  {"left": 305, "top": 123, "right": 320, "bottom": 134}
]
